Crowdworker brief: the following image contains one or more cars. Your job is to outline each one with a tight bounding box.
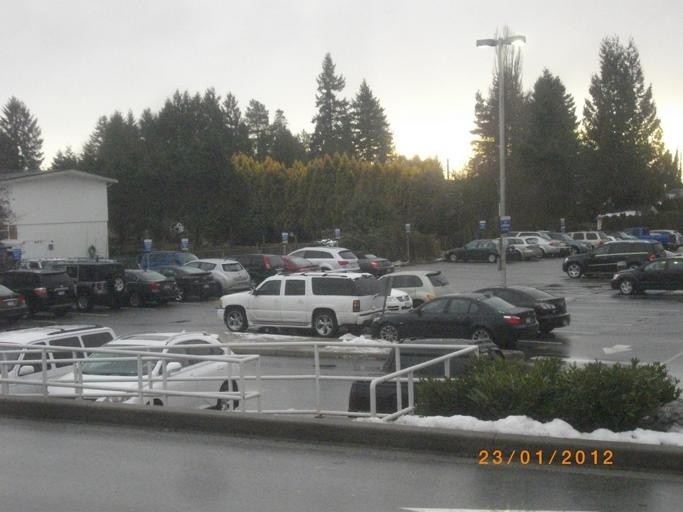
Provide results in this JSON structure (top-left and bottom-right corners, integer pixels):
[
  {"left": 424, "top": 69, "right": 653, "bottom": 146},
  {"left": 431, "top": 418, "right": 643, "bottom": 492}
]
[
  {"left": 42, "top": 332, "right": 245, "bottom": 412},
  {"left": 381, "top": 288, "right": 413, "bottom": 313},
  {"left": 0, "top": 286, "right": 29, "bottom": 324},
  {"left": 376, "top": 269, "right": 454, "bottom": 305},
  {"left": 183, "top": 258, "right": 251, "bottom": 297},
  {"left": 441, "top": 227, "right": 682, "bottom": 263},
  {"left": 368, "top": 292, "right": 540, "bottom": 348},
  {"left": 610, "top": 257, "right": 682, "bottom": 297},
  {"left": 347, "top": 338, "right": 524, "bottom": 413},
  {"left": 120, "top": 267, "right": 177, "bottom": 308},
  {"left": 353, "top": 250, "right": 393, "bottom": 279},
  {"left": 281, "top": 255, "right": 319, "bottom": 273},
  {"left": 473, "top": 285, "right": 569, "bottom": 338},
  {"left": 153, "top": 264, "right": 218, "bottom": 303}
]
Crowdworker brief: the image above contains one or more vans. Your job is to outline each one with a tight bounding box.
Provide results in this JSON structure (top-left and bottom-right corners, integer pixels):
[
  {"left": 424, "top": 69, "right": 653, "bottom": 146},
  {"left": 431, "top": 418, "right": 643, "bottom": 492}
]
[{"left": 138, "top": 250, "right": 198, "bottom": 273}]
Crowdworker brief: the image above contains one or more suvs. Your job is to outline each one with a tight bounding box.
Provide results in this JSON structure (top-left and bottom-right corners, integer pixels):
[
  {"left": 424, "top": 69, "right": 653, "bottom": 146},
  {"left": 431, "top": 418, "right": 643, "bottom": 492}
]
[
  {"left": 225, "top": 254, "right": 285, "bottom": 292},
  {"left": 561, "top": 239, "right": 666, "bottom": 279},
  {"left": 41, "top": 261, "right": 128, "bottom": 314},
  {"left": 215, "top": 270, "right": 386, "bottom": 339},
  {"left": 0, "top": 323, "right": 116, "bottom": 399},
  {"left": 0, "top": 269, "right": 77, "bottom": 319},
  {"left": 287, "top": 245, "right": 360, "bottom": 274}
]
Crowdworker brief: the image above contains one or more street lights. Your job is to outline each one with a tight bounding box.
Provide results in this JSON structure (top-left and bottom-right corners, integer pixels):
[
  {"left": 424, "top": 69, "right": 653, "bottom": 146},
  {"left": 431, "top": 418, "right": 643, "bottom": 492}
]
[{"left": 475, "top": 33, "right": 526, "bottom": 287}]
